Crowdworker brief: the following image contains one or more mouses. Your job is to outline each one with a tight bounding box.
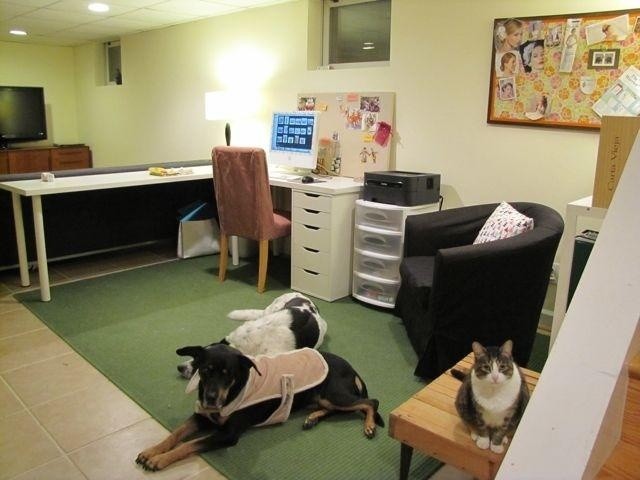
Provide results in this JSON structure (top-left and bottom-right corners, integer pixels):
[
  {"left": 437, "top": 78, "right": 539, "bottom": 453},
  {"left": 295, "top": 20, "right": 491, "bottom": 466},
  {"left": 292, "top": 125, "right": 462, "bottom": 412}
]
[{"left": 302, "top": 176, "right": 313, "bottom": 183}]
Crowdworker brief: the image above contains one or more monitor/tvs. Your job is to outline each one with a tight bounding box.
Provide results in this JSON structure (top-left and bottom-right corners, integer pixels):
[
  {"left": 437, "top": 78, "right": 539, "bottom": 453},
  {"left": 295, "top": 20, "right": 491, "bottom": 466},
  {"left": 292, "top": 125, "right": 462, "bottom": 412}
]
[
  {"left": 0, "top": 86, "right": 47, "bottom": 149},
  {"left": 269, "top": 110, "right": 320, "bottom": 180}
]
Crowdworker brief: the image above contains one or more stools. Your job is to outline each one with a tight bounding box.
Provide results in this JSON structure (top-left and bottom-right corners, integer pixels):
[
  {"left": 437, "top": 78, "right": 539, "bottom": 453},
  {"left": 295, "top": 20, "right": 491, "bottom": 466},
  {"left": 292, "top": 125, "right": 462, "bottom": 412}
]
[{"left": 388, "top": 344, "right": 545, "bottom": 480}]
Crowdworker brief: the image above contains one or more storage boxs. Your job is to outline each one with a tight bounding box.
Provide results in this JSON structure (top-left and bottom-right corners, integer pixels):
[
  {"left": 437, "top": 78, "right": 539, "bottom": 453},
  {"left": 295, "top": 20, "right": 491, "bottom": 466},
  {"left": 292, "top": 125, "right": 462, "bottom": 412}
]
[{"left": 591, "top": 111, "right": 640, "bottom": 211}]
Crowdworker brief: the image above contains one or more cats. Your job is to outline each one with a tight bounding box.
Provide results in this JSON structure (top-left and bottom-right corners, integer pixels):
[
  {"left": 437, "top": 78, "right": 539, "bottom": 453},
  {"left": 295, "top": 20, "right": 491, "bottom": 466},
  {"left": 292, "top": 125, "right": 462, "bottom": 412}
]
[{"left": 445, "top": 339, "right": 530, "bottom": 453}]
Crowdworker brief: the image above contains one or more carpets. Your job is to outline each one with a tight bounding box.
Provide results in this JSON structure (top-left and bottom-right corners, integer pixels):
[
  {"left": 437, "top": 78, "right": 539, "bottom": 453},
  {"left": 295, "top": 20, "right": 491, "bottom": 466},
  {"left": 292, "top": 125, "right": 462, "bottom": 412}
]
[{"left": 10, "top": 252, "right": 550, "bottom": 480}]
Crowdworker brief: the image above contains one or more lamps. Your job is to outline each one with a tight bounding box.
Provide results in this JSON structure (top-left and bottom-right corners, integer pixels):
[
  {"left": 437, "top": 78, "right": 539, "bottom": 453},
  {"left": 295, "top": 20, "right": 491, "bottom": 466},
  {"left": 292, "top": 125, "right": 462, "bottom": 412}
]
[{"left": 202, "top": 88, "right": 244, "bottom": 150}]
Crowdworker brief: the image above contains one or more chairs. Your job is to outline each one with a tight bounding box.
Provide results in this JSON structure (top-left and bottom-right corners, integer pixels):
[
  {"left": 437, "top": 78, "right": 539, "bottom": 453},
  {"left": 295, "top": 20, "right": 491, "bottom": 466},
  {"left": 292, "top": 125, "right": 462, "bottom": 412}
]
[
  {"left": 210, "top": 144, "right": 293, "bottom": 295},
  {"left": 391, "top": 194, "right": 564, "bottom": 384}
]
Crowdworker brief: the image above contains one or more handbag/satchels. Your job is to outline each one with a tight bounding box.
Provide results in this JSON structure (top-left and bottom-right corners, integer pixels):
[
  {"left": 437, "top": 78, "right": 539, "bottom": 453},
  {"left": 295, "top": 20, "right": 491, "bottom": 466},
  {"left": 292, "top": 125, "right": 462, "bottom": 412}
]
[{"left": 175, "top": 200, "right": 222, "bottom": 260}]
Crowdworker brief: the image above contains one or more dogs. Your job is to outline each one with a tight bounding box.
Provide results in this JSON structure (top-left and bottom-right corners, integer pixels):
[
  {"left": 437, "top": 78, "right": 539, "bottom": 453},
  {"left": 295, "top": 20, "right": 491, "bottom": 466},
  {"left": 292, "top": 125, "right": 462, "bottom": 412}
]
[
  {"left": 176, "top": 291, "right": 328, "bottom": 379},
  {"left": 134, "top": 345, "right": 385, "bottom": 472}
]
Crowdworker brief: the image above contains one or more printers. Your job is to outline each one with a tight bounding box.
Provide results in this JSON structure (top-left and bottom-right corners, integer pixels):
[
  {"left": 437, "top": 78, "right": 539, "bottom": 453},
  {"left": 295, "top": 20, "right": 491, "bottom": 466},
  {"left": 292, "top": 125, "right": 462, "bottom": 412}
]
[{"left": 364, "top": 170, "right": 441, "bottom": 206}]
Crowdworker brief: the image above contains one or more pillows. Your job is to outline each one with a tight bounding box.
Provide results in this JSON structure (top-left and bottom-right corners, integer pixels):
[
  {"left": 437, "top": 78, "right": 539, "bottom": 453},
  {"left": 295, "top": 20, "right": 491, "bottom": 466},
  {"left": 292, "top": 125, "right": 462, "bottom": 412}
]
[{"left": 473, "top": 197, "right": 534, "bottom": 246}]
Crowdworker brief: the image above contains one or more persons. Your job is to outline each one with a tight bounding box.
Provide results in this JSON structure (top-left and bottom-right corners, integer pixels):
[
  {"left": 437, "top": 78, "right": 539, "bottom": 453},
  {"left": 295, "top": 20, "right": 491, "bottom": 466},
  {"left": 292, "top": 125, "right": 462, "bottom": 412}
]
[
  {"left": 500, "top": 52, "right": 517, "bottom": 74},
  {"left": 602, "top": 24, "right": 621, "bottom": 41},
  {"left": 500, "top": 82, "right": 514, "bottom": 99},
  {"left": 522, "top": 41, "right": 545, "bottom": 71},
  {"left": 564, "top": 27, "right": 577, "bottom": 71},
  {"left": 498, "top": 19, "right": 523, "bottom": 51}
]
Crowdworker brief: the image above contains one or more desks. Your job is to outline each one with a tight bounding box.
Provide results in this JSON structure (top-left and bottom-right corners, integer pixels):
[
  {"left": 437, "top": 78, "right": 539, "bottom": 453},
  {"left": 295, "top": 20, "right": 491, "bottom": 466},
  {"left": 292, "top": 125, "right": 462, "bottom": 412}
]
[
  {"left": 226, "top": 167, "right": 363, "bottom": 301},
  {"left": 543, "top": 192, "right": 614, "bottom": 365},
  {"left": 0, "top": 163, "right": 216, "bottom": 303}
]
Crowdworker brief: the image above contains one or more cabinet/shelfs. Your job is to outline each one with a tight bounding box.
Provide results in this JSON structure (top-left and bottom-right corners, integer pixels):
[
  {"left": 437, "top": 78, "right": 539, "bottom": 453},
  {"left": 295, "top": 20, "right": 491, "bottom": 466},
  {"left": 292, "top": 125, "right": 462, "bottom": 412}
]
[
  {"left": 352, "top": 199, "right": 443, "bottom": 315},
  {"left": 0, "top": 143, "right": 94, "bottom": 176}
]
